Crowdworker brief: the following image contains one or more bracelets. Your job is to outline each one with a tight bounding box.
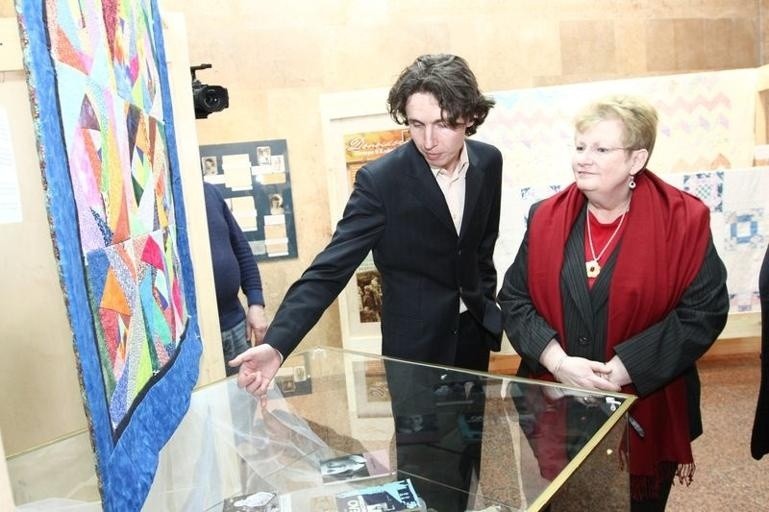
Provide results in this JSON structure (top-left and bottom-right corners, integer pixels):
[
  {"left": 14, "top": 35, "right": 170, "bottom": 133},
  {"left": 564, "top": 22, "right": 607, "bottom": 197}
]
[{"left": 553, "top": 354, "right": 570, "bottom": 382}]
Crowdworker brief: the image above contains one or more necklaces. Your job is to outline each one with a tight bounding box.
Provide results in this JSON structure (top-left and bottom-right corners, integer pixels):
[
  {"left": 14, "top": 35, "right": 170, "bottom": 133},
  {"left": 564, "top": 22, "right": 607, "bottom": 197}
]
[{"left": 585, "top": 191, "right": 632, "bottom": 278}]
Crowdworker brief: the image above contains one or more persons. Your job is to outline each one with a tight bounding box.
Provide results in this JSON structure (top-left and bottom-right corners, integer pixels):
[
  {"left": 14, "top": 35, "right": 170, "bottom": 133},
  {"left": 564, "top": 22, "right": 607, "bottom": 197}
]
[
  {"left": 321, "top": 455, "right": 368, "bottom": 476},
  {"left": 269, "top": 194, "right": 284, "bottom": 214},
  {"left": 203, "top": 182, "right": 268, "bottom": 376},
  {"left": 497, "top": 93, "right": 730, "bottom": 512},
  {"left": 226, "top": 56, "right": 504, "bottom": 512},
  {"left": 204, "top": 158, "right": 215, "bottom": 176},
  {"left": 751, "top": 244, "right": 768, "bottom": 460}
]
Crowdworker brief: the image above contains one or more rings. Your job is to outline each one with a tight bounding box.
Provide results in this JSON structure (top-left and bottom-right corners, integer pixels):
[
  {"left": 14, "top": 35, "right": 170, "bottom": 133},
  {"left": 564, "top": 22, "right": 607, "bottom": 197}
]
[{"left": 587, "top": 392, "right": 593, "bottom": 399}]
